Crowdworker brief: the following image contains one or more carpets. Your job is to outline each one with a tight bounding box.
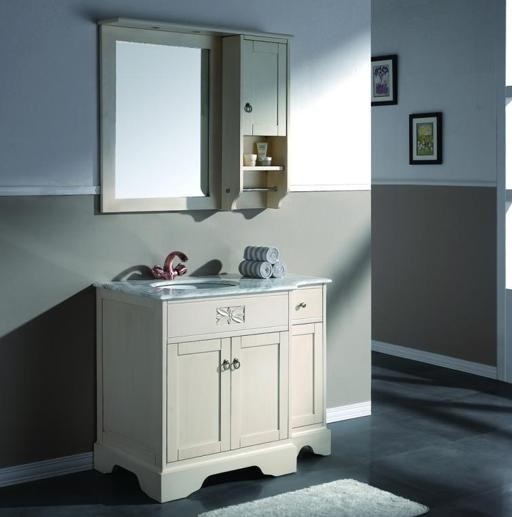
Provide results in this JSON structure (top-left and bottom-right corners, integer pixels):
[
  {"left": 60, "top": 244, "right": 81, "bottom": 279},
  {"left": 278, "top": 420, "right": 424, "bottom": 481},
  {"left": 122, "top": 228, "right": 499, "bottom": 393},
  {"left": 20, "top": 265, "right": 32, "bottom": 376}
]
[{"left": 196, "top": 479, "right": 430, "bottom": 517}]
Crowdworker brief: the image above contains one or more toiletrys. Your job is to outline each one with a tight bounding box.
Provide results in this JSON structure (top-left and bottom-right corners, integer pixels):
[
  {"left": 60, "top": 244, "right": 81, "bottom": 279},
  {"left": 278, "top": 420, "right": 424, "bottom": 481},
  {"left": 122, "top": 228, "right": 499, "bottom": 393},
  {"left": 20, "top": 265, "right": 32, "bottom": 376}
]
[
  {"left": 244, "top": 152, "right": 257, "bottom": 166},
  {"left": 255, "top": 141, "right": 268, "bottom": 165},
  {"left": 260, "top": 156, "right": 273, "bottom": 166}
]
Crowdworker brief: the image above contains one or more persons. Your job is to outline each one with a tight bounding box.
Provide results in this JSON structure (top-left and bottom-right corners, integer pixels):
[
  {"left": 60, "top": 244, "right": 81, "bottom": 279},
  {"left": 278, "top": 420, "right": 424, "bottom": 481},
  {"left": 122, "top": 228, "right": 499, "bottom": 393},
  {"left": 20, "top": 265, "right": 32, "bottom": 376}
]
[{"left": 415, "top": 135, "right": 433, "bottom": 151}]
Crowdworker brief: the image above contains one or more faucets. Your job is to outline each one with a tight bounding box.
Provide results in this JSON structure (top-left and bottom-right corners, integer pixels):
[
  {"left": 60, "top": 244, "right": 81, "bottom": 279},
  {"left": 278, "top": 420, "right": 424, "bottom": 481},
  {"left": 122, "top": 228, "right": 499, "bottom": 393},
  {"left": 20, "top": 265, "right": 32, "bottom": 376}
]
[{"left": 162, "top": 250, "right": 188, "bottom": 281}]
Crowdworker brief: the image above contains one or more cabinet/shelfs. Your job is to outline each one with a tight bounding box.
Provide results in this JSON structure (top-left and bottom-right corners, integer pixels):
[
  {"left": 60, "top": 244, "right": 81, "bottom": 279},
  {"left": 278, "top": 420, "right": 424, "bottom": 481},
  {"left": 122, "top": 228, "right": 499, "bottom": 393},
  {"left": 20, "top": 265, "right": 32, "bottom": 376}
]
[
  {"left": 93, "top": 275, "right": 333, "bottom": 503},
  {"left": 223, "top": 30, "right": 294, "bottom": 210}
]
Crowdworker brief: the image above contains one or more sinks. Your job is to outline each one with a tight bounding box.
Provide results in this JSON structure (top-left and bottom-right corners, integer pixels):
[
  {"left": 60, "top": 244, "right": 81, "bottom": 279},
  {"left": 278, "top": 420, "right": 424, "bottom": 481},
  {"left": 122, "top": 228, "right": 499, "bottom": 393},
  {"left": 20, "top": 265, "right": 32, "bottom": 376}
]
[{"left": 150, "top": 278, "right": 240, "bottom": 289}]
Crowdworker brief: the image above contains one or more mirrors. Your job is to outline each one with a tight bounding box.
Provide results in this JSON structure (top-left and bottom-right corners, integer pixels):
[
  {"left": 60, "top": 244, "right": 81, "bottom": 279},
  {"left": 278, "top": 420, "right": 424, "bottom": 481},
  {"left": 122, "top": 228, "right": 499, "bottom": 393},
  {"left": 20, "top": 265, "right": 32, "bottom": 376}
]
[{"left": 99, "top": 25, "right": 223, "bottom": 214}]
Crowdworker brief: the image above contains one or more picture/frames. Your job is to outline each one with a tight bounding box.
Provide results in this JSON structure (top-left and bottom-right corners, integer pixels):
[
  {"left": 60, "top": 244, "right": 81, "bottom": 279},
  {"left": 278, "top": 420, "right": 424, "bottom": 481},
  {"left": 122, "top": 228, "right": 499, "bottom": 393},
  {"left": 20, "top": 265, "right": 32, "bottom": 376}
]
[
  {"left": 409, "top": 112, "right": 442, "bottom": 165},
  {"left": 371, "top": 55, "right": 398, "bottom": 106}
]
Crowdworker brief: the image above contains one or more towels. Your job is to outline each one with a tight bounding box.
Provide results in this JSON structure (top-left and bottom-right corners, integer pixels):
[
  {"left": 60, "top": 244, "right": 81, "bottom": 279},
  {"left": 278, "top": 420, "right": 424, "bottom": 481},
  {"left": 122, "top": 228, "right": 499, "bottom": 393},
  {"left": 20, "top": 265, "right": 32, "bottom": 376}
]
[{"left": 239, "top": 246, "right": 286, "bottom": 278}]
